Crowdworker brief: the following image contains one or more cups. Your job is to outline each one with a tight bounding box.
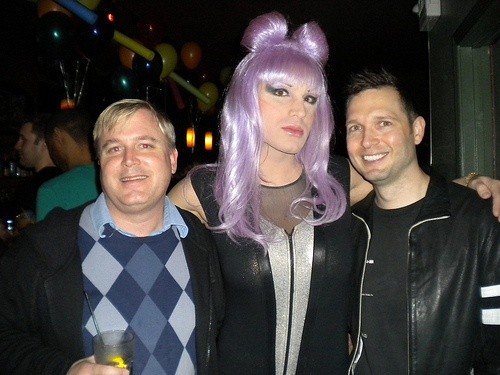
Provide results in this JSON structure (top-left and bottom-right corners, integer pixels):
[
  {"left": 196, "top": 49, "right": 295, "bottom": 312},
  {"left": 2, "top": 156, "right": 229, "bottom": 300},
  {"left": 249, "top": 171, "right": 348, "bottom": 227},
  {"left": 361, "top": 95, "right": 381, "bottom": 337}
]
[
  {"left": 5, "top": 162, "right": 27, "bottom": 177},
  {"left": 93, "top": 329, "right": 134, "bottom": 375}
]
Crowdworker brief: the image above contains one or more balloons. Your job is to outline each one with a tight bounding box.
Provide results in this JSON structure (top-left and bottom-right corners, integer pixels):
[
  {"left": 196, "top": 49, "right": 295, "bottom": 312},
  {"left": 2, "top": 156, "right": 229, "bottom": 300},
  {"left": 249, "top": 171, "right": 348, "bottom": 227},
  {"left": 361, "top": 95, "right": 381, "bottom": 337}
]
[
  {"left": 182, "top": 41, "right": 201, "bottom": 70},
  {"left": 155, "top": 43, "right": 177, "bottom": 80},
  {"left": 38, "top": 11, "right": 73, "bottom": 48},
  {"left": 119, "top": 39, "right": 145, "bottom": 70},
  {"left": 85, "top": 7, "right": 116, "bottom": 43},
  {"left": 197, "top": 80, "right": 219, "bottom": 112},
  {"left": 131, "top": 47, "right": 162, "bottom": 78},
  {"left": 106, "top": 68, "right": 146, "bottom": 102},
  {"left": 37, "top": 0, "right": 72, "bottom": 17},
  {"left": 76, "top": 0, "right": 101, "bottom": 10},
  {"left": 134, "top": 70, "right": 163, "bottom": 104},
  {"left": 137, "top": 6, "right": 170, "bottom": 50}
]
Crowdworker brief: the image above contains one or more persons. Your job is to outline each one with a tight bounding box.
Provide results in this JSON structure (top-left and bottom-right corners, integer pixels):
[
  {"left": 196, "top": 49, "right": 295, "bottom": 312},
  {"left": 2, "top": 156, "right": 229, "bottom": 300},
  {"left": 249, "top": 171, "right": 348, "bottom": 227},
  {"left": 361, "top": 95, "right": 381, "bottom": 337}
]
[
  {"left": 0, "top": 120, "right": 68, "bottom": 215},
  {"left": 341, "top": 66, "right": 500, "bottom": 375},
  {"left": 0, "top": 98, "right": 228, "bottom": 375},
  {"left": 36, "top": 106, "right": 102, "bottom": 223},
  {"left": 168, "top": 13, "right": 500, "bottom": 375}
]
